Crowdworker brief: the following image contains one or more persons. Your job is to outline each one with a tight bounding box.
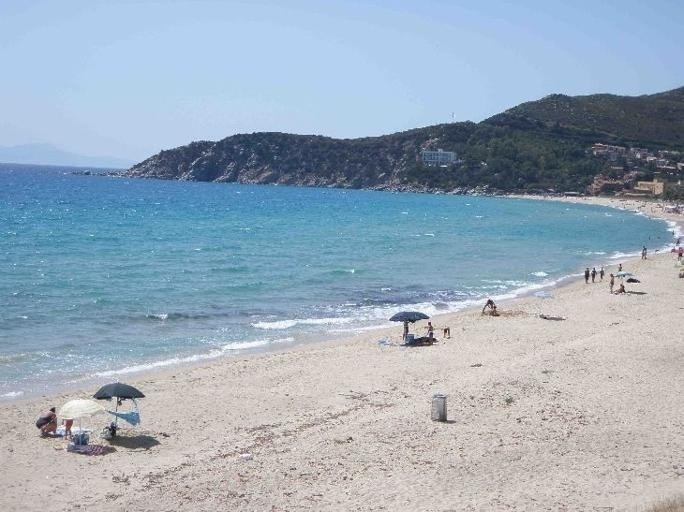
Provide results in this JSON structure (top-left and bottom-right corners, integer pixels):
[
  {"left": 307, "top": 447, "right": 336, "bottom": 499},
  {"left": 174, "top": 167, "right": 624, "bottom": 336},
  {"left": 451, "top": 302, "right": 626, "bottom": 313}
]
[
  {"left": 482, "top": 298, "right": 500, "bottom": 316},
  {"left": 402, "top": 321, "right": 412, "bottom": 340},
  {"left": 61, "top": 419, "right": 74, "bottom": 440},
  {"left": 423, "top": 321, "right": 433, "bottom": 342},
  {"left": 584, "top": 229, "right": 684, "bottom": 294},
  {"left": 440, "top": 323, "right": 451, "bottom": 339},
  {"left": 35, "top": 406, "right": 57, "bottom": 438}
]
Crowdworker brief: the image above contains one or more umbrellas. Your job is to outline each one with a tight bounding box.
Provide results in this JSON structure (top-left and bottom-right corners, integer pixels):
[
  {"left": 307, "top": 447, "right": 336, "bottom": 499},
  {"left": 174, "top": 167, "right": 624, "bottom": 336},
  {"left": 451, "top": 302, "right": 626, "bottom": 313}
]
[
  {"left": 389, "top": 311, "right": 430, "bottom": 333},
  {"left": 93, "top": 381, "right": 145, "bottom": 421},
  {"left": 58, "top": 399, "right": 105, "bottom": 446}
]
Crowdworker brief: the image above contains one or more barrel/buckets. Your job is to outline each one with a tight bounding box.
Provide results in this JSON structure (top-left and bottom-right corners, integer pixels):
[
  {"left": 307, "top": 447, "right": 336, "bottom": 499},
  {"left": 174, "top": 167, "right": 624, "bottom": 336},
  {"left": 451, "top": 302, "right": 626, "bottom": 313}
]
[
  {"left": 405, "top": 334, "right": 414, "bottom": 344},
  {"left": 431, "top": 395, "right": 447, "bottom": 421}
]
